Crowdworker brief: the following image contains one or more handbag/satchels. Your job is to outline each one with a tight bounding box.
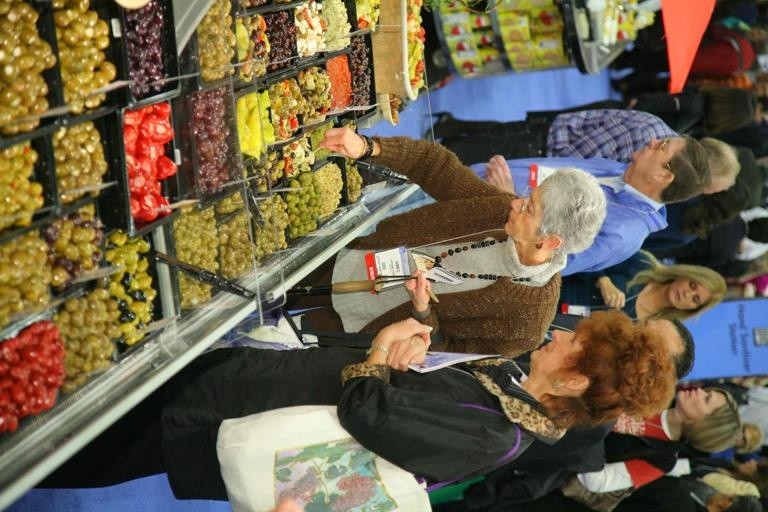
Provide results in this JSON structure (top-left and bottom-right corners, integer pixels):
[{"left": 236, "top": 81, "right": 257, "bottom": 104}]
[
  {"left": 208, "top": 307, "right": 443, "bottom": 352},
  {"left": 215, "top": 401, "right": 521, "bottom": 512}
]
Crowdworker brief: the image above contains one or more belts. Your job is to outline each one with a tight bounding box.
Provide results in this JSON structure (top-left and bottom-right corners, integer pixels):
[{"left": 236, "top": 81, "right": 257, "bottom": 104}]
[{"left": 528, "top": 123, "right": 545, "bottom": 157}]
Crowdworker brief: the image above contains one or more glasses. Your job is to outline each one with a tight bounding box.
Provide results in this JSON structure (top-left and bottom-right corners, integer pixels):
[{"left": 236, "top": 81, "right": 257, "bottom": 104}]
[{"left": 653, "top": 137, "right": 673, "bottom": 174}]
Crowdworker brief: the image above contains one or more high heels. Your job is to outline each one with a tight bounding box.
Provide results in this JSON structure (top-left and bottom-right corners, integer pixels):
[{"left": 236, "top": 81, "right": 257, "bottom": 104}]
[{"left": 423, "top": 110, "right": 452, "bottom": 144}]
